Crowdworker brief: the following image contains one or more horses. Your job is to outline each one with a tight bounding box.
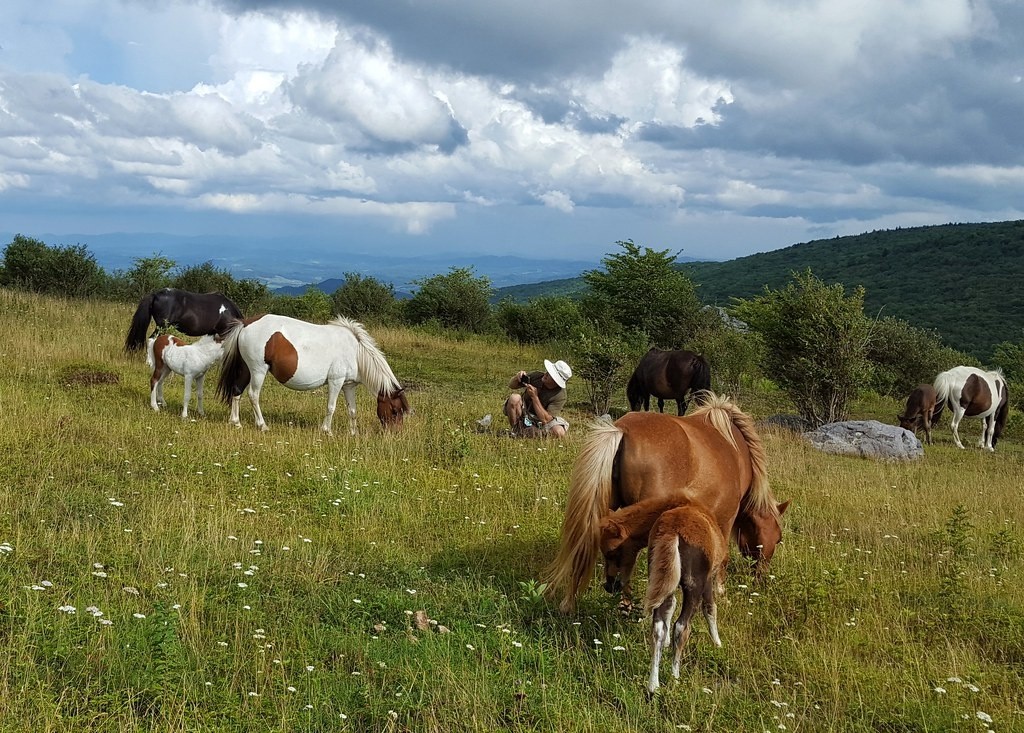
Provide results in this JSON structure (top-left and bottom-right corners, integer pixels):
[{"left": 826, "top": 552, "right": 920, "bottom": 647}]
[
  {"left": 119, "top": 286, "right": 415, "bottom": 441},
  {"left": 896, "top": 364, "right": 1011, "bottom": 454},
  {"left": 535, "top": 346, "right": 793, "bottom": 694}
]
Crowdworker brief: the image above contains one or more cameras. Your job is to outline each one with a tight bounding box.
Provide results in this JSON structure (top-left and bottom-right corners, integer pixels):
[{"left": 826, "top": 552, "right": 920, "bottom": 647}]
[{"left": 520, "top": 375, "right": 530, "bottom": 384}]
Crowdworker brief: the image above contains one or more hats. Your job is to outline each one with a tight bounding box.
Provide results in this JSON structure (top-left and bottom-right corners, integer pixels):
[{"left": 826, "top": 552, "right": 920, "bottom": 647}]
[{"left": 544, "top": 359, "right": 572, "bottom": 389}]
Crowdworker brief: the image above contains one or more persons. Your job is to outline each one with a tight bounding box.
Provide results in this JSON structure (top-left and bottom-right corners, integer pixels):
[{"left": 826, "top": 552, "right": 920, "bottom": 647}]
[{"left": 502, "top": 359, "right": 572, "bottom": 438}]
